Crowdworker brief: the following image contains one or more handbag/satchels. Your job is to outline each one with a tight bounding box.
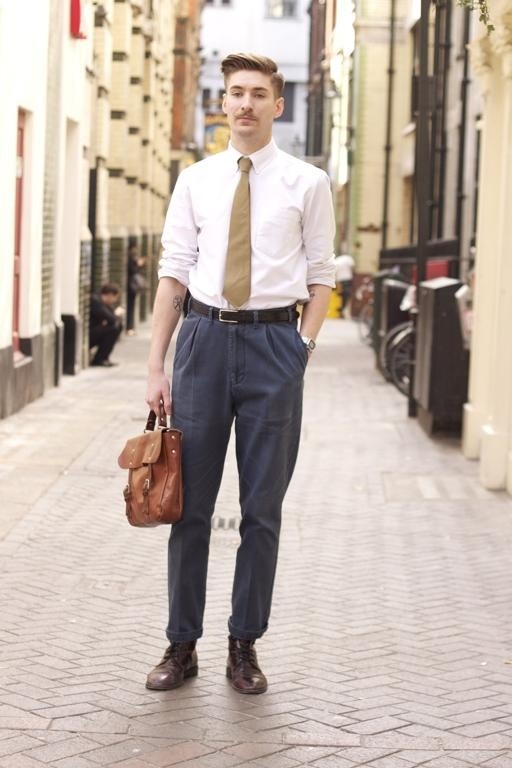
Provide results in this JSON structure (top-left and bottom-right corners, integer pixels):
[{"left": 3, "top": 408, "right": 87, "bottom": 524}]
[{"left": 119, "top": 403, "right": 182, "bottom": 527}]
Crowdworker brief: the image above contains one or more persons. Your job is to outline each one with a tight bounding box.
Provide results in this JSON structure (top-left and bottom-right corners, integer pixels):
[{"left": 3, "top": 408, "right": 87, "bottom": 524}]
[
  {"left": 88, "top": 284, "right": 127, "bottom": 366},
  {"left": 126, "top": 235, "right": 151, "bottom": 336},
  {"left": 332, "top": 246, "right": 358, "bottom": 319},
  {"left": 143, "top": 51, "right": 339, "bottom": 699}
]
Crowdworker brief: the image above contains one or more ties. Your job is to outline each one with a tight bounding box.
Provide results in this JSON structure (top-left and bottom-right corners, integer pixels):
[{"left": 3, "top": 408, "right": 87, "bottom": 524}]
[{"left": 222, "top": 158, "right": 251, "bottom": 308}]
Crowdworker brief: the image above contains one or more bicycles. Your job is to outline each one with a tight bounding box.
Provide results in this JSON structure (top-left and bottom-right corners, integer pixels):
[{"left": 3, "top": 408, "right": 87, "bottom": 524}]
[{"left": 356, "top": 275, "right": 418, "bottom": 395}]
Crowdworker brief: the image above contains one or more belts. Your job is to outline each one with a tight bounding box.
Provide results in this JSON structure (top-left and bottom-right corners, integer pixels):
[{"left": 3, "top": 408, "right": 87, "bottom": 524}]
[{"left": 190, "top": 299, "right": 300, "bottom": 325}]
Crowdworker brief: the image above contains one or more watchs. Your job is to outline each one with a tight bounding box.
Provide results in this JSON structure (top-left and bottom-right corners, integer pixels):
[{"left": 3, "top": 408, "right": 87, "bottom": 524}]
[{"left": 298, "top": 335, "right": 317, "bottom": 356}]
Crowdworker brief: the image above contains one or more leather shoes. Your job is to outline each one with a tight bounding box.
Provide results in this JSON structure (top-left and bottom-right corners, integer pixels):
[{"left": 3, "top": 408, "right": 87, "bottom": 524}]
[
  {"left": 145, "top": 640, "right": 198, "bottom": 692},
  {"left": 226, "top": 635, "right": 268, "bottom": 693}
]
